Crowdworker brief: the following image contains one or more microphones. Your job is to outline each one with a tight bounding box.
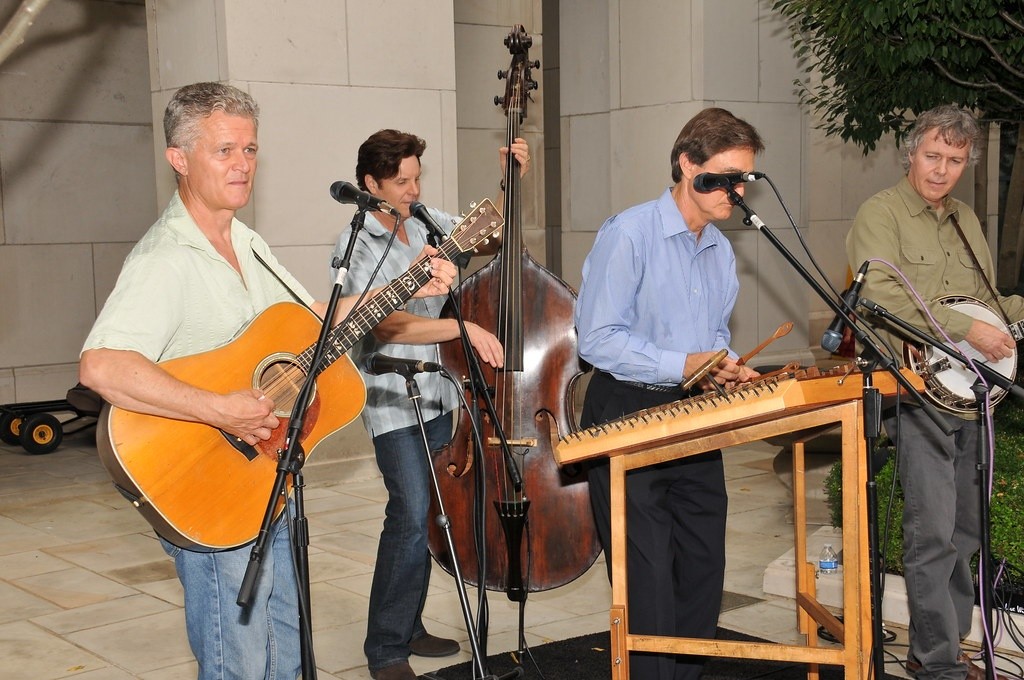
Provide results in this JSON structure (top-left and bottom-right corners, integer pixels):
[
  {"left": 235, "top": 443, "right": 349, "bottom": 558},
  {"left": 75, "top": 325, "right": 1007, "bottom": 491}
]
[
  {"left": 409, "top": 200, "right": 448, "bottom": 242},
  {"left": 328, "top": 180, "right": 399, "bottom": 216},
  {"left": 819, "top": 259, "right": 870, "bottom": 354},
  {"left": 692, "top": 171, "right": 766, "bottom": 194}
]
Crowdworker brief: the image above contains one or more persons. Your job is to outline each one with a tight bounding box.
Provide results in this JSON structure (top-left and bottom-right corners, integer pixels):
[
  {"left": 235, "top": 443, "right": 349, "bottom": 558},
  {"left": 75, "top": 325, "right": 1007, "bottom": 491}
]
[
  {"left": 575, "top": 107, "right": 767, "bottom": 680},
  {"left": 329, "top": 129, "right": 531, "bottom": 680},
  {"left": 844, "top": 106, "right": 1023, "bottom": 679},
  {"left": 78, "top": 82, "right": 458, "bottom": 680}
]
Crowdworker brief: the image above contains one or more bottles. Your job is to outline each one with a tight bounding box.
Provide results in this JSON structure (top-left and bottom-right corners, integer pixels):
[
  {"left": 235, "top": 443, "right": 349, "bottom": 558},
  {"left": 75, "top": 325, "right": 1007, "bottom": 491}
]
[{"left": 819, "top": 542, "right": 839, "bottom": 575}]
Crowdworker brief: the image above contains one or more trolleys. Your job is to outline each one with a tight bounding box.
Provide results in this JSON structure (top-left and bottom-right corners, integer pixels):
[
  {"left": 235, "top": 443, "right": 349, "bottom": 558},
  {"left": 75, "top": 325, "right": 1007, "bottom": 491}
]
[{"left": 5, "top": 381, "right": 128, "bottom": 454}]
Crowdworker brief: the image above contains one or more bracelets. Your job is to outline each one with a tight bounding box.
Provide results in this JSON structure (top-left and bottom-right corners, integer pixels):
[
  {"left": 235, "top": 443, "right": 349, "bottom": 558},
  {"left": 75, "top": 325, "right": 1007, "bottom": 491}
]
[{"left": 500, "top": 180, "right": 506, "bottom": 191}]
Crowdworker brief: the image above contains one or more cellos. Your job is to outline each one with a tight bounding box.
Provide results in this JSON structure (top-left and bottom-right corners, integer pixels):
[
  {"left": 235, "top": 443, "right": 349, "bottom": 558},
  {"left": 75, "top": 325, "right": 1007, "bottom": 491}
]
[{"left": 422, "top": 18, "right": 606, "bottom": 680}]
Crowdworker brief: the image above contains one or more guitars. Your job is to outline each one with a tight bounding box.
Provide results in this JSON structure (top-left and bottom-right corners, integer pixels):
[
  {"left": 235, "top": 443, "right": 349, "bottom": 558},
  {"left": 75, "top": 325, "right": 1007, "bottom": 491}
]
[
  {"left": 94, "top": 192, "right": 510, "bottom": 557},
  {"left": 908, "top": 292, "right": 1024, "bottom": 415}
]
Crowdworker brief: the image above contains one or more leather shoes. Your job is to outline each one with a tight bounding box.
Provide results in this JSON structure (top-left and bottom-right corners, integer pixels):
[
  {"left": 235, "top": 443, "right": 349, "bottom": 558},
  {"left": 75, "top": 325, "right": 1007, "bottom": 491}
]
[{"left": 906, "top": 653, "right": 1009, "bottom": 680}]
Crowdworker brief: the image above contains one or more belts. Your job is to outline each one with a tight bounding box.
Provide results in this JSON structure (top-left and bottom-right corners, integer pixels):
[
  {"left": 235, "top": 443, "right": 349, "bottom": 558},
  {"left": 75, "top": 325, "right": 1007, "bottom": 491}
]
[{"left": 623, "top": 380, "right": 680, "bottom": 393}]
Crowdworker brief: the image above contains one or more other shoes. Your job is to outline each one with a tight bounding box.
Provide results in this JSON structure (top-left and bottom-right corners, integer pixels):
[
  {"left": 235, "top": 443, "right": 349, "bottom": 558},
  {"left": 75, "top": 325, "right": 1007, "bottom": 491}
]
[
  {"left": 370, "top": 663, "right": 418, "bottom": 680},
  {"left": 409, "top": 634, "right": 460, "bottom": 657}
]
